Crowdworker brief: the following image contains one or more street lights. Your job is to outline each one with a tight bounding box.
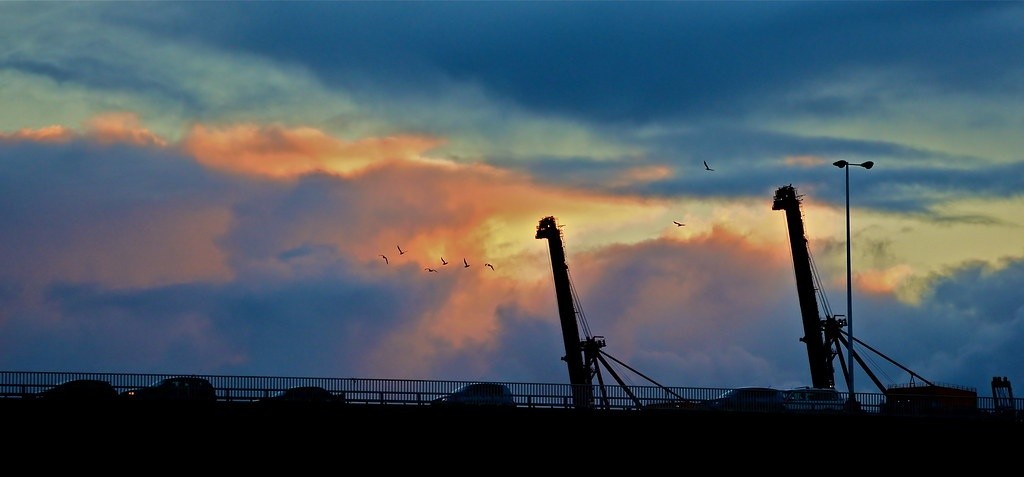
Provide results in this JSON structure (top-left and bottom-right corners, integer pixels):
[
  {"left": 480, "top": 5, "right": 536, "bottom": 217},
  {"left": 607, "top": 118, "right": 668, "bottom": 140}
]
[{"left": 832, "top": 159, "right": 876, "bottom": 388}]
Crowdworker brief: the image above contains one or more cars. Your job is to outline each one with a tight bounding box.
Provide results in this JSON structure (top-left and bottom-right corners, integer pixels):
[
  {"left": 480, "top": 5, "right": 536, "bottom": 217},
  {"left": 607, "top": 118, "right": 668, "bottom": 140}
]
[
  {"left": 428, "top": 383, "right": 517, "bottom": 408},
  {"left": 709, "top": 385, "right": 846, "bottom": 415},
  {"left": 22, "top": 378, "right": 119, "bottom": 401},
  {"left": 119, "top": 377, "right": 219, "bottom": 401},
  {"left": 271, "top": 385, "right": 345, "bottom": 403}
]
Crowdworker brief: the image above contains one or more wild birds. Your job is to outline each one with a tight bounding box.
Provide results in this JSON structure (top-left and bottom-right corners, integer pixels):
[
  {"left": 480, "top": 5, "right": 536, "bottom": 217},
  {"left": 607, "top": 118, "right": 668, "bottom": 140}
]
[
  {"left": 703, "top": 160, "right": 715, "bottom": 172},
  {"left": 462, "top": 257, "right": 471, "bottom": 269},
  {"left": 377, "top": 244, "right": 408, "bottom": 265},
  {"left": 672, "top": 220, "right": 685, "bottom": 228},
  {"left": 423, "top": 256, "right": 449, "bottom": 273},
  {"left": 484, "top": 263, "right": 495, "bottom": 272}
]
